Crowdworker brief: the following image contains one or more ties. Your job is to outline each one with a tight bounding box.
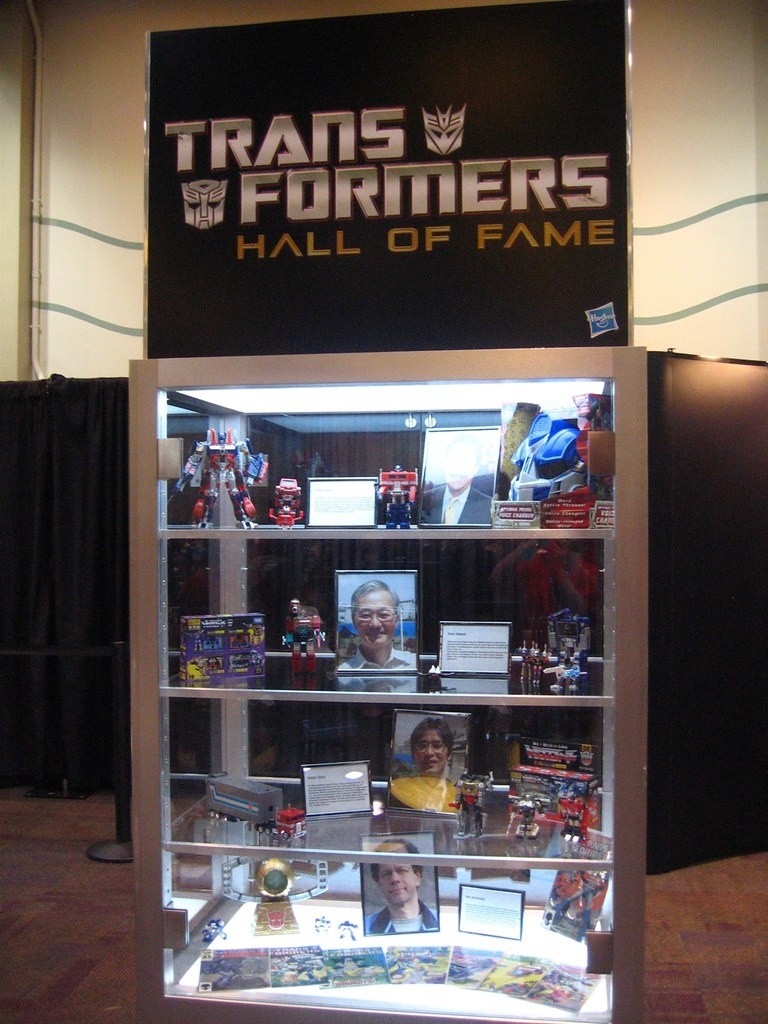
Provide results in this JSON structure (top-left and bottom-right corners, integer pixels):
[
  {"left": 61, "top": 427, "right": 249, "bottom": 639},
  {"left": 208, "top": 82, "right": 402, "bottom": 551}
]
[{"left": 443, "top": 497, "right": 458, "bottom": 524}]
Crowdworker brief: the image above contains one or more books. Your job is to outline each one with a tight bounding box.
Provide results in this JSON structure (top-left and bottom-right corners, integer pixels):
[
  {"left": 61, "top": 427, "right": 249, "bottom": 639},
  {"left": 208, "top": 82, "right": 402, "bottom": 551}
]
[{"left": 199, "top": 947, "right": 601, "bottom": 1014}]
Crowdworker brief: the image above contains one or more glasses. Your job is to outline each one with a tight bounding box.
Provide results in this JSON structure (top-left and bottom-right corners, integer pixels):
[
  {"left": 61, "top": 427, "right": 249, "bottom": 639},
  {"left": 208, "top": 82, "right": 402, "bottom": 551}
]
[
  {"left": 380, "top": 867, "right": 413, "bottom": 880},
  {"left": 354, "top": 610, "right": 397, "bottom": 621},
  {"left": 415, "top": 742, "right": 447, "bottom": 754}
]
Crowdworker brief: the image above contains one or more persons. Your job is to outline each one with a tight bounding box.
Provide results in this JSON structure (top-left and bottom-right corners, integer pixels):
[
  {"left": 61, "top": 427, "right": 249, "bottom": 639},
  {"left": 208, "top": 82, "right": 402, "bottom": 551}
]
[
  {"left": 422, "top": 438, "right": 494, "bottom": 524},
  {"left": 201, "top": 918, "right": 227, "bottom": 942},
  {"left": 449, "top": 774, "right": 588, "bottom": 845},
  {"left": 410, "top": 718, "right": 466, "bottom": 787},
  {"left": 514, "top": 639, "right": 549, "bottom": 685},
  {"left": 492, "top": 538, "right": 604, "bottom": 656},
  {"left": 364, "top": 839, "right": 437, "bottom": 936},
  {"left": 281, "top": 600, "right": 326, "bottom": 675},
  {"left": 175, "top": 428, "right": 418, "bottom": 530},
  {"left": 338, "top": 580, "right": 416, "bottom": 670}
]
